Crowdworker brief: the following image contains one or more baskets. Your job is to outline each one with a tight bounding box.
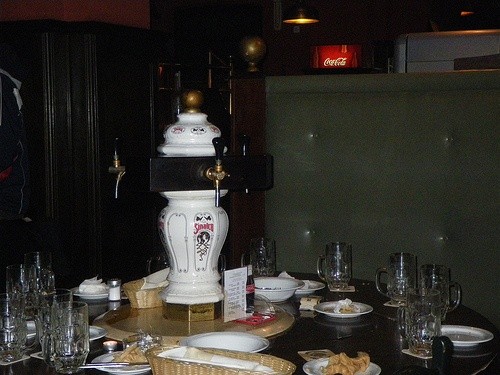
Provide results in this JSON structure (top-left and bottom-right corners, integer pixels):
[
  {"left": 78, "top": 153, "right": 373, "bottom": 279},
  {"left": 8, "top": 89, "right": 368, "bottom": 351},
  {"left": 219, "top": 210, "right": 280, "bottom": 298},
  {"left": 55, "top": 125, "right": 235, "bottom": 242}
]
[
  {"left": 146, "top": 348, "right": 297, "bottom": 375},
  {"left": 123, "top": 280, "right": 169, "bottom": 308}
]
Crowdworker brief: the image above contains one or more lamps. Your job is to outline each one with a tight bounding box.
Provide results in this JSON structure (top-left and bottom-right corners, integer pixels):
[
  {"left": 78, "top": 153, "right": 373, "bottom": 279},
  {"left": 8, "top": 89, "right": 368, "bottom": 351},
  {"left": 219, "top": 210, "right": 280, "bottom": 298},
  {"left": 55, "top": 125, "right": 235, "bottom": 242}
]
[{"left": 282, "top": 0, "right": 319, "bottom": 25}]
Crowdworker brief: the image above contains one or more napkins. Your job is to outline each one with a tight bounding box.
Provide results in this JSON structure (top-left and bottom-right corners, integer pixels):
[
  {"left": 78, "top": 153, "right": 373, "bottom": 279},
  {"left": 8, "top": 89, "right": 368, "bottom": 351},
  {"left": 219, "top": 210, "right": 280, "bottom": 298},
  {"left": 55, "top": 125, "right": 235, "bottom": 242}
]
[
  {"left": 79, "top": 275, "right": 109, "bottom": 293},
  {"left": 278, "top": 271, "right": 310, "bottom": 290}
]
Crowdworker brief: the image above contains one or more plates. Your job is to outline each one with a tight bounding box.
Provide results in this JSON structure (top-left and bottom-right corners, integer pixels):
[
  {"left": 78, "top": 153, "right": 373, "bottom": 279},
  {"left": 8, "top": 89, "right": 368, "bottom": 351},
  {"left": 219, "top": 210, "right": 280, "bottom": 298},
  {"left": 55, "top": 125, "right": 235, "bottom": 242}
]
[
  {"left": 294, "top": 280, "right": 325, "bottom": 296},
  {"left": 303, "top": 358, "right": 381, "bottom": 375},
  {"left": 313, "top": 301, "right": 373, "bottom": 318},
  {"left": 442, "top": 324, "right": 494, "bottom": 351},
  {"left": 91, "top": 351, "right": 152, "bottom": 375},
  {"left": 179, "top": 331, "right": 270, "bottom": 353},
  {"left": 88, "top": 325, "right": 108, "bottom": 343},
  {"left": 69, "top": 286, "right": 108, "bottom": 301}
]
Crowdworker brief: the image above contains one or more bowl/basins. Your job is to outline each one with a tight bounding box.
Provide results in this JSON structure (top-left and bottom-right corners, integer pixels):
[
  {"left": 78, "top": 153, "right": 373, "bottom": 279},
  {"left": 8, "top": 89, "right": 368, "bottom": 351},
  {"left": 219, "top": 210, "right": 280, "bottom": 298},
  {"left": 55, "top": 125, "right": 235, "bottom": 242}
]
[{"left": 253, "top": 277, "right": 305, "bottom": 302}]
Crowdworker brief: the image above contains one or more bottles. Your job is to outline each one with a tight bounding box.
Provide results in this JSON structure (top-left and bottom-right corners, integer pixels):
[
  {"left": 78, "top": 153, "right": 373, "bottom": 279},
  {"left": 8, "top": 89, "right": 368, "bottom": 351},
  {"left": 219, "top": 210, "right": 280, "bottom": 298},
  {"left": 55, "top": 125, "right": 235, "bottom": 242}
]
[
  {"left": 107, "top": 280, "right": 121, "bottom": 301},
  {"left": 103, "top": 340, "right": 117, "bottom": 354},
  {"left": 161, "top": 91, "right": 227, "bottom": 157},
  {"left": 246, "top": 265, "right": 256, "bottom": 311},
  {"left": 158, "top": 189, "right": 228, "bottom": 305}
]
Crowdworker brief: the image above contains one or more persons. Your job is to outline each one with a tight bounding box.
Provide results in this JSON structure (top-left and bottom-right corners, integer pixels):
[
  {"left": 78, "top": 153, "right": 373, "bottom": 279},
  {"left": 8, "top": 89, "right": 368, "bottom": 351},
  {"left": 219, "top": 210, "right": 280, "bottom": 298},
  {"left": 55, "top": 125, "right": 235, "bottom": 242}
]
[{"left": 0, "top": 69, "right": 33, "bottom": 297}]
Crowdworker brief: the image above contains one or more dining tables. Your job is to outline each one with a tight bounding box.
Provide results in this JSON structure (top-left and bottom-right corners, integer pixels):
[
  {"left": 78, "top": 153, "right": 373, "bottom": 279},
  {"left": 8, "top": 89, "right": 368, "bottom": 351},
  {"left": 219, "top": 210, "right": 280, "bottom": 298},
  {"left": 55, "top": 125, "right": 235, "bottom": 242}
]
[{"left": 0, "top": 270, "right": 500, "bottom": 375}]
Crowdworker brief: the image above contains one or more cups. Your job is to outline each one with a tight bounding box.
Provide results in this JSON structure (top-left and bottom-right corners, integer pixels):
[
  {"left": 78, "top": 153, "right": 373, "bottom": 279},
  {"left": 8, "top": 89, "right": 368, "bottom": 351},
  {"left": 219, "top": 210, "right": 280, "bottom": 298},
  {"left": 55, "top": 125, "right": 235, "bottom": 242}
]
[
  {"left": 38, "top": 289, "right": 73, "bottom": 367},
  {"left": 51, "top": 302, "right": 90, "bottom": 375},
  {"left": 241, "top": 238, "right": 275, "bottom": 278},
  {"left": 417, "top": 263, "right": 462, "bottom": 322},
  {"left": 316, "top": 241, "right": 351, "bottom": 292},
  {"left": 399, "top": 287, "right": 442, "bottom": 356},
  {"left": 147, "top": 245, "right": 226, "bottom": 286},
  {"left": 0, "top": 251, "right": 55, "bottom": 363},
  {"left": 375, "top": 252, "right": 416, "bottom": 308}
]
